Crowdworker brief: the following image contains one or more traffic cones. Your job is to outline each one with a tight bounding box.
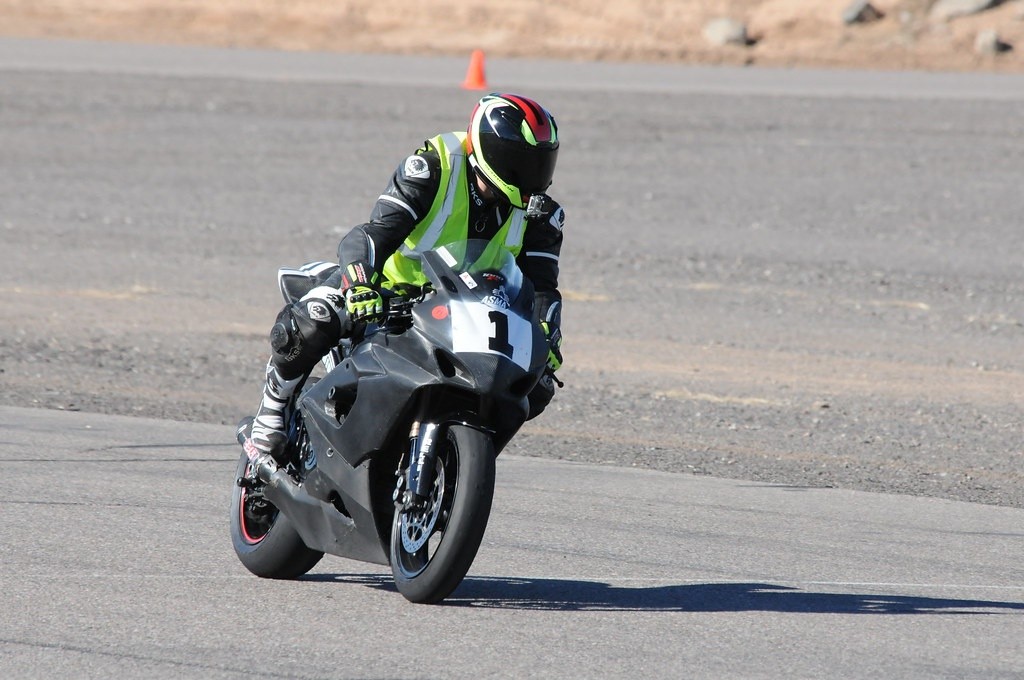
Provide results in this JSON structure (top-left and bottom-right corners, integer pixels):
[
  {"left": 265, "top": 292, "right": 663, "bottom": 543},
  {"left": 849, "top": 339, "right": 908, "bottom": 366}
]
[{"left": 461, "top": 47, "right": 487, "bottom": 94}]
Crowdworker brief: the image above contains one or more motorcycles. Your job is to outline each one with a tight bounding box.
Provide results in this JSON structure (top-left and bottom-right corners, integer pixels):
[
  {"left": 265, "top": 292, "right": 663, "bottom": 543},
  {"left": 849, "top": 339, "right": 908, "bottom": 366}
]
[{"left": 229, "top": 237, "right": 567, "bottom": 605}]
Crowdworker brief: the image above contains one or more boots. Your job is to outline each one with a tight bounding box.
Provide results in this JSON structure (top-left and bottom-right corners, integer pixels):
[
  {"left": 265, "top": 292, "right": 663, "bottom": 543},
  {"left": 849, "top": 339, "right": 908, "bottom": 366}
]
[{"left": 250, "top": 354, "right": 304, "bottom": 454}]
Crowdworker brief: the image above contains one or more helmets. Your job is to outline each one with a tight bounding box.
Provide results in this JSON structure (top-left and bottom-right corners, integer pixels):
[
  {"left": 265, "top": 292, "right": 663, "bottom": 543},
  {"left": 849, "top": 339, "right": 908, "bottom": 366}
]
[{"left": 466, "top": 92, "right": 560, "bottom": 208}]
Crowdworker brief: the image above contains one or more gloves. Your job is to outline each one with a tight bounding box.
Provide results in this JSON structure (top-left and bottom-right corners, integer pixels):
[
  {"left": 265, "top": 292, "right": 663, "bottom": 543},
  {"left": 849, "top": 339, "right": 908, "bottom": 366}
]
[
  {"left": 539, "top": 321, "right": 563, "bottom": 374},
  {"left": 345, "top": 286, "right": 384, "bottom": 326}
]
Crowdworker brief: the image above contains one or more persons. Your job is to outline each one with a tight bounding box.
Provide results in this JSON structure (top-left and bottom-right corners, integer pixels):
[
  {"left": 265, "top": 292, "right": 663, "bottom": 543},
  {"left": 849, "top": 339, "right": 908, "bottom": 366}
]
[{"left": 251, "top": 92, "right": 565, "bottom": 453}]
